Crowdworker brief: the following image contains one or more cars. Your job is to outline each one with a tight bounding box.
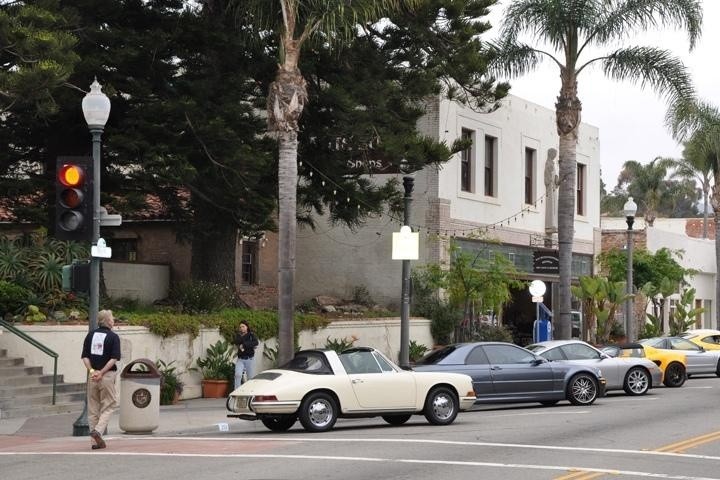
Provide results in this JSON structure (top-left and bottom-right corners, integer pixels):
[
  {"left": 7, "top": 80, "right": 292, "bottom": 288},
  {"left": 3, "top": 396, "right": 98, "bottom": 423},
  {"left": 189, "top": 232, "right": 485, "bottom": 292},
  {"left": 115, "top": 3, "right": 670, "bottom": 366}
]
[
  {"left": 638, "top": 336, "right": 720, "bottom": 378},
  {"left": 523, "top": 340, "right": 663, "bottom": 395},
  {"left": 409, "top": 342, "right": 606, "bottom": 408},
  {"left": 683, "top": 329, "right": 720, "bottom": 350}
]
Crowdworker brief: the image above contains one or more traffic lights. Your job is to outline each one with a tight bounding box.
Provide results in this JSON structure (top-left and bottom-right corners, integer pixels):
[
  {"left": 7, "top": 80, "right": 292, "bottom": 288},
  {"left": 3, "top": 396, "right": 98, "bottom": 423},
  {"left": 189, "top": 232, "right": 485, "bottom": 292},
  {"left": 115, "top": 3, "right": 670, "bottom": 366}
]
[{"left": 54, "top": 156, "right": 92, "bottom": 240}]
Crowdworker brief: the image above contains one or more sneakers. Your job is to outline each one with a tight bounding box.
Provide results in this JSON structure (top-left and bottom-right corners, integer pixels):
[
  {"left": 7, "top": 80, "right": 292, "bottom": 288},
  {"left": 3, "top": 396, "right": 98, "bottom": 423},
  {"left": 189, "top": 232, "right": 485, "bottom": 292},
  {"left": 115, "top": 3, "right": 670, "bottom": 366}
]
[{"left": 89, "top": 430, "right": 106, "bottom": 449}]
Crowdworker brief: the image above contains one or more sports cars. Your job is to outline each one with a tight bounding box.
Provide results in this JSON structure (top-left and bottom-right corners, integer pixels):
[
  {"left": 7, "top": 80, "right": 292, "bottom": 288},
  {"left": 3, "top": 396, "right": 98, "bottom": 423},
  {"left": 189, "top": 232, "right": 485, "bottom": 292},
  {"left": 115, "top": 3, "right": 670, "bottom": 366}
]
[
  {"left": 601, "top": 343, "right": 688, "bottom": 394},
  {"left": 226, "top": 345, "right": 476, "bottom": 432}
]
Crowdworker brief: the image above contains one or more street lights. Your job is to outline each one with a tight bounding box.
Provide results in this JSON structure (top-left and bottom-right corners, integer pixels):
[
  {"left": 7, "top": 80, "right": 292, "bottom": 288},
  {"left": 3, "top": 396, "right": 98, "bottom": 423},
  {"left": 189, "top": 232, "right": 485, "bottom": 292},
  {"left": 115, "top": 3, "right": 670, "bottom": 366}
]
[
  {"left": 623, "top": 196, "right": 638, "bottom": 344},
  {"left": 390, "top": 175, "right": 420, "bottom": 371},
  {"left": 529, "top": 280, "right": 547, "bottom": 343},
  {"left": 72, "top": 75, "right": 114, "bottom": 436}
]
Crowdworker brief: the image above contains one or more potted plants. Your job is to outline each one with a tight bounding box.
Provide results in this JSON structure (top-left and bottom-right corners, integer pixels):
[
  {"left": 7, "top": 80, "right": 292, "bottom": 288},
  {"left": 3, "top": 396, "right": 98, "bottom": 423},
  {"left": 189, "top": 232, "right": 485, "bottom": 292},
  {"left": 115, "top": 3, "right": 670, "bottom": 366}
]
[
  {"left": 136, "top": 356, "right": 184, "bottom": 406},
  {"left": 188, "top": 339, "right": 238, "bottom": 398}
]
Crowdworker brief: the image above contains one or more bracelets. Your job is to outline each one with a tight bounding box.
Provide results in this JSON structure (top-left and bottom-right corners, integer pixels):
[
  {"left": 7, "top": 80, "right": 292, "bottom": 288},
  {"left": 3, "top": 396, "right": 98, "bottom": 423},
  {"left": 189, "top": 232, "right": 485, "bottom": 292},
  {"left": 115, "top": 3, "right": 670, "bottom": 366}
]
[{"left": 88, "top": 367, "right": 96, "bottom": 375}]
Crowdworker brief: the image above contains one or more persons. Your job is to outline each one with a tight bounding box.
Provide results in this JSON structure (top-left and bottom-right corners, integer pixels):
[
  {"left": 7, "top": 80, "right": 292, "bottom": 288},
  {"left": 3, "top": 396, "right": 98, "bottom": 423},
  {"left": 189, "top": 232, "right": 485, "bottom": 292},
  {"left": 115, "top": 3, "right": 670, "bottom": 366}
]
[
  {"left": 233, "top": 318, "right": 258, "bottom": 390},
  {"left": 79, "top": 308, "right": 124, "bottom": 450}
]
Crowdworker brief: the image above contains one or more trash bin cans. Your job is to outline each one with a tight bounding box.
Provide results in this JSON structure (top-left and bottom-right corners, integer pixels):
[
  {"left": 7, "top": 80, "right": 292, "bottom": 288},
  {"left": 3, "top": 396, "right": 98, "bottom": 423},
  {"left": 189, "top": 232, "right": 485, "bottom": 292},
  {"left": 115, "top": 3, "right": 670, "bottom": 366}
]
[{"left": 119, "top": 358, "right": 161, "bottom": 435}]
